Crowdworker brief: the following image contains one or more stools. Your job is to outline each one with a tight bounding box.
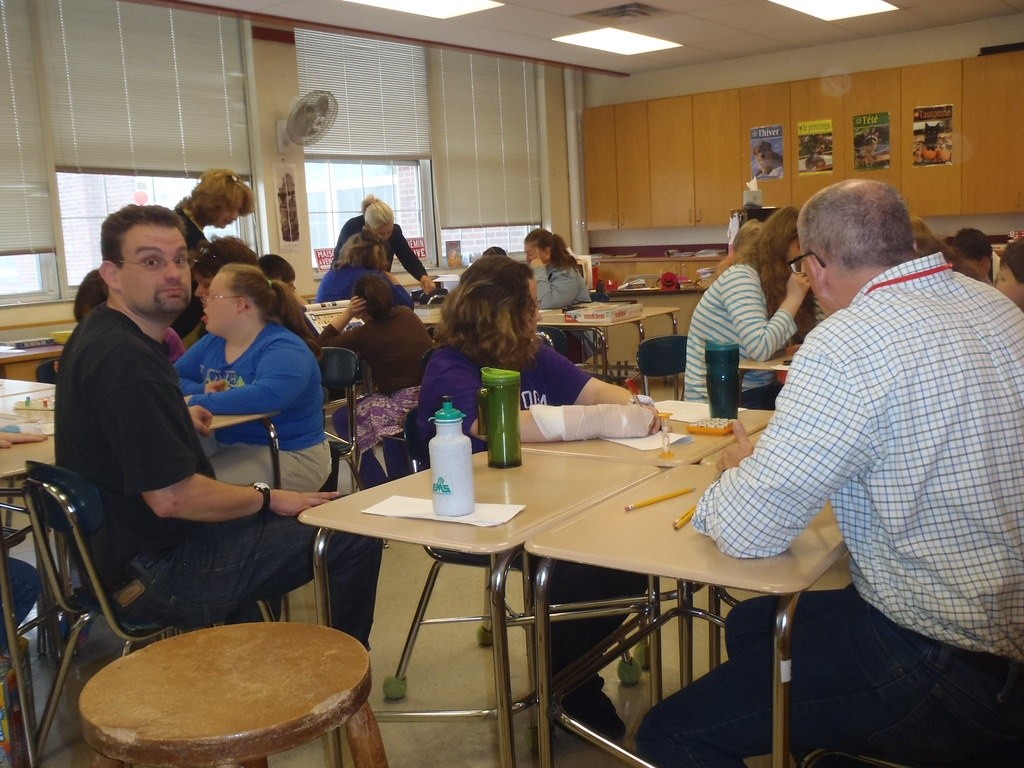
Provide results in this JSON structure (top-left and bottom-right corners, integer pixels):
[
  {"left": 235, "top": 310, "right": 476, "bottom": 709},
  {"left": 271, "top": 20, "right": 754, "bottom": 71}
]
[{"left": 78, "top": 621, "right": 390, "bottom": 768}]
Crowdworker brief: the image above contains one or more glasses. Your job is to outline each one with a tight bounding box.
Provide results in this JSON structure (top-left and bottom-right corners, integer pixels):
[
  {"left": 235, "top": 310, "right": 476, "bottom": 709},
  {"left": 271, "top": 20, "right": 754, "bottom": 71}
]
[
  {"left": 524, "top": 298, "right": 542, "bottom": 314},
  {"left": 198, "top": 246, "right": 221, "bottom": 260},
  {"left": 114, "top": 252, "right": 198, "bottom": 276},
  {"left": 225, "top": 175, "right": 238, "bottom": 185},
  {"left": 788, "top": 251, "right": 826, "bottom": 275},
  {"left": 200, "top": 293, "right": 250, "bottom": 311}
]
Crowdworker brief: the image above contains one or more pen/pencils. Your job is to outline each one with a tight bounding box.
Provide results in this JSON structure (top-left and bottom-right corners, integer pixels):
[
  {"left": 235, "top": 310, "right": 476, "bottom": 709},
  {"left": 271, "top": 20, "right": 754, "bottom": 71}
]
[
  {"left": 625, "top": 378, "right": 642, "bottom": 406},
  {"left": 673, "top": 505, "right": 697, "bottom": 530},
  {"left": 624, "top": 487, "right": 696, "bottom": 511}
]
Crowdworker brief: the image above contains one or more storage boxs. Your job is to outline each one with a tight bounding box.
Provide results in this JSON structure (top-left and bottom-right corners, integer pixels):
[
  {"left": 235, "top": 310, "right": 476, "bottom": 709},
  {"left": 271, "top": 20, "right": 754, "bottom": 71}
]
[
  {"left": 565, "top": 302, "right": 642, "bottom": 324},
  {"left": 0, "top": 636, "right": 39, "bottom": 768}
]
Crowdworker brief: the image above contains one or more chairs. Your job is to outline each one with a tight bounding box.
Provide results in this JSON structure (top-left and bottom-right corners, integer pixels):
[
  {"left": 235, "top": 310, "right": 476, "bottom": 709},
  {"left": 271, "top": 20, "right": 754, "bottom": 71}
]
[
  {"left": 319, "top": 347, "right": 652, "bottom": 754},
  {"left": 37, "top": 357, "right": 60, "bottom": 384},
  {"left": 637, "top": 335, "right": 688, "bottom": 401},
  {"left": 21, "top": 458, "right": 341, "bottom": 768},
  {"left": 586, "top": 291, "right": 608, "bottom": 371},
  {"left": 535, "top": 324, "right": 568, "bottom": 356}
]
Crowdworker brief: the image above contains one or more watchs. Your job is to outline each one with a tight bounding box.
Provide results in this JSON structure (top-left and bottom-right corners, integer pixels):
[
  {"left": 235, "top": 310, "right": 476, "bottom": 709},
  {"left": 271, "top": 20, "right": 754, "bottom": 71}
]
[{"left": 250, "top": 481, "right": 271, "bottom": 514}]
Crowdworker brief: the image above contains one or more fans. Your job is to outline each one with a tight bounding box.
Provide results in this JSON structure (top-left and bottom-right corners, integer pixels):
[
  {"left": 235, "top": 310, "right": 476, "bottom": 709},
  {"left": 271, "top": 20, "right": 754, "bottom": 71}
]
[{"left": 275, "top": 89, "right": 338, "bottom": 163}]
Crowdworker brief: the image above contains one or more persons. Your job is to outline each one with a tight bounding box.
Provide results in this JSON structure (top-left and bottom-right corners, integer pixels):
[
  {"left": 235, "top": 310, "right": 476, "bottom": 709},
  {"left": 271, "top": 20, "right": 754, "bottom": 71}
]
[
  {"left": 54, "top": 168, "right": 383, "bottom": 650},
  {"left": 315, "top": 192, "right": 437, "bottom": 488},
  {"left": 0, "top": 433, "right": 48, "bottom": 657},
  {"left": 636, "top": 178, "right": 1024, "bottom": 767},
  {"left": 524, "top": 228, "right": 595, "bottom": 364},
  {"left": 413, "top": 256, "right": 663, "bottom": 738},
  {"left": 483, "top": 247, "right": 506, "bottom": 255}
]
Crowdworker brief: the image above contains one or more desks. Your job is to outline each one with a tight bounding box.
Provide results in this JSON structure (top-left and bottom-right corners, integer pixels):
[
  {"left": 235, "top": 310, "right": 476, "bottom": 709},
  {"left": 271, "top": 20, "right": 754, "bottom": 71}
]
[
  {"left": 297, "top": 401, "right": 849, "bottom": 767},
  {"left": 736, "top": 342, "right": 802, "bottom": 403},
  {"left": 0, "top": 379, "right": 283, "bottom": 768},
  {"left": 0, "top": 344, "right": 64, "bottom": 382},
  {"left": 414, "top": 283, "right": 710, "bottom": 396}
]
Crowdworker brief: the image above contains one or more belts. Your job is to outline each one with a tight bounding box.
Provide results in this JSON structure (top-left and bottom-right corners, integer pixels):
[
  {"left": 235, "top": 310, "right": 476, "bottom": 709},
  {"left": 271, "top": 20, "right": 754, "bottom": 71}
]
[{"left": 910, "top": 628, "right": 1024, "bottom": 684}]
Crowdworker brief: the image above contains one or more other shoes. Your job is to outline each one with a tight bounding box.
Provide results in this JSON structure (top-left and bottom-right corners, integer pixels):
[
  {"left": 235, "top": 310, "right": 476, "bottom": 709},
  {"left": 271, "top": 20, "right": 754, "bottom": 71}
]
[
  {"left": 555, "top": 684, "right": 626, "bottom": 746},
  {"left": 57, "top": 618, "right": 91, "bottom": 644}
]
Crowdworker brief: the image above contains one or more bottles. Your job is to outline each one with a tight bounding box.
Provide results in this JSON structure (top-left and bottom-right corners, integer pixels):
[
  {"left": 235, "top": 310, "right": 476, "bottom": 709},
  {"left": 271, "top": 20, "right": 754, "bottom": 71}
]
[
  {"left": 477, "top": 366, "right": 523, "bottom": 469},
  {"left": 428, "top": 396, "right": 476, "bottom": 517},
  {"left": 705, "top": 341, "right": 739, "bottom": 420}
]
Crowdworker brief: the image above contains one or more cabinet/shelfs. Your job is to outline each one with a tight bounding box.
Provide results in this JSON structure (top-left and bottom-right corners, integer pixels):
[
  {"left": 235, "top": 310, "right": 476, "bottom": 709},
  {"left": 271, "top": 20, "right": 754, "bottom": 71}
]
[
  {"left": 598, "top": 261, "right": 722, "bottom": 283},
  {"left": 583, "top": 49, "right": 1024, "bottom": 231}
]
